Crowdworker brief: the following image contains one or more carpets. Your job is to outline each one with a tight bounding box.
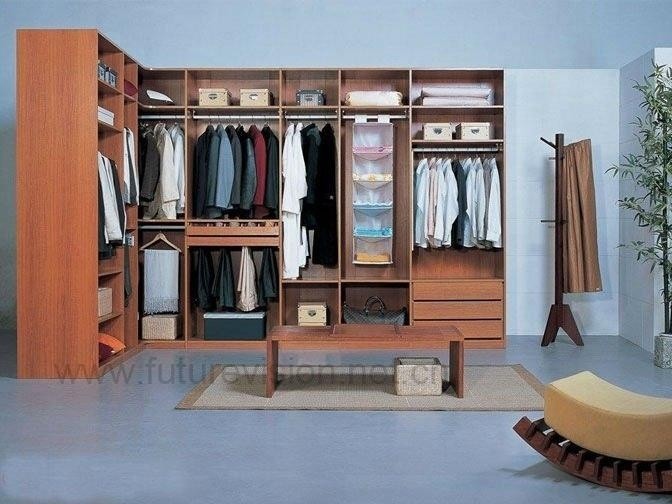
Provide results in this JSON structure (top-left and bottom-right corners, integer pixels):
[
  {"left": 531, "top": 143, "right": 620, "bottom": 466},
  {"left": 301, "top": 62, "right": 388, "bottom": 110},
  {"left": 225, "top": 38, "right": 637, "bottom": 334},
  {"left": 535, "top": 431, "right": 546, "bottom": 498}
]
[{"left": 171, "top": 361, "right": 550, "bottom": 417}]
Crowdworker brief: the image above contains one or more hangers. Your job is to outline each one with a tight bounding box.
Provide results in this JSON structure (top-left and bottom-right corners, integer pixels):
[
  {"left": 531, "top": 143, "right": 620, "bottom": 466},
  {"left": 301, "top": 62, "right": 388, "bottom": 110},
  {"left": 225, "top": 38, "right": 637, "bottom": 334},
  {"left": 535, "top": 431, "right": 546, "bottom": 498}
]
[
  {"left": 135, "top": 114, "right": 329, "bottom": 132},
  {"left": 420, "top": 146, "right": 497, "bottom": 162},
  {"left": 138, "top": 227, "right": 183, "bottom": 255}
]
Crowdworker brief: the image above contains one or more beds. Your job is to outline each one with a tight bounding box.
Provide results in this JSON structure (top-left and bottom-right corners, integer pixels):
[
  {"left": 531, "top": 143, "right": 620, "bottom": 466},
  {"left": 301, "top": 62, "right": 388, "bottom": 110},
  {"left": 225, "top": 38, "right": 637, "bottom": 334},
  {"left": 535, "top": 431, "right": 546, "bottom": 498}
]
[{"left": 262, "top": 322, "right": 466, "bottom": 399}]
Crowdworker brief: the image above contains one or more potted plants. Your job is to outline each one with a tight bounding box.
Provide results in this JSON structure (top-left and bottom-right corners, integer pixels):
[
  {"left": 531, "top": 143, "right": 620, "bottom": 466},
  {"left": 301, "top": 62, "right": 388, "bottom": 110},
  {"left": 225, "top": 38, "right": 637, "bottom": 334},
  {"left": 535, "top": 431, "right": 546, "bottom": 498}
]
[{"left": 604, "top": 55, "right": 672, "bottom": 372}]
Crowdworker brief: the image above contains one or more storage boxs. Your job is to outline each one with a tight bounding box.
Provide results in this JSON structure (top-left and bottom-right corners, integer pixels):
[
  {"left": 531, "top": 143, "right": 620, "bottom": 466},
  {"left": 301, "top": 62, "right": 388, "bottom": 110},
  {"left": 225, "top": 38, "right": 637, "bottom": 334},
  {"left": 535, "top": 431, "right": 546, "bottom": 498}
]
[
  {"left": 96, "top": 59, "right": 119, "bottom": 126},
  {"left": 392, "top": 356, "right": 444, "bottom": 397},
  {"left": 202, "top": 309, "right": 266, "bottom": 341},
  {"left": 295, "top": 88, "right": 327, "bottom": 108},
  {"left": 295, "top": 300, "right": 329, "bottom": 326},
  {"left": 97, "top": 287, "right": 112, "bottom": 317},
  {"left": 197, "top": 86, "right": 233, "bottom": 107},
  {"left": 422, "top": 122, "right": 454, "bottom": 141},
  {"left": 141, "top": 314, "right": 182, "bottom": 340},
  {"left": 455, "top": 120, "right": 492, "bottom": 140},
  {"left": 239, "top": 88, "right": 274, "bottom": 107}
]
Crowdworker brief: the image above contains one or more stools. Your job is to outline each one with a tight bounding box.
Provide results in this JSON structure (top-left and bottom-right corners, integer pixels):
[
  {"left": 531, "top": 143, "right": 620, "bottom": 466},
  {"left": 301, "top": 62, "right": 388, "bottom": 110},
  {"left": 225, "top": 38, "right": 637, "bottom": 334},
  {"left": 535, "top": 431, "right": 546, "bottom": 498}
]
[{"left": 511, "top": 369, "right": 672, "bottom": 495}]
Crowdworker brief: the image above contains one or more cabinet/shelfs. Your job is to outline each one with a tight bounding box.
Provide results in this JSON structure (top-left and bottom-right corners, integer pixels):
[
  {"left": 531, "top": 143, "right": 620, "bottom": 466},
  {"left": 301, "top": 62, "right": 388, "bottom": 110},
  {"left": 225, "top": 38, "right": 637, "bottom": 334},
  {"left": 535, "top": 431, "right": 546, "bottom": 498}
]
[
  {"left": 187, "top": 67, "right": 282, "bottom": 352},
  {"left": 122, "top": 52, "right": 138, "bottom": 359},
  {"left": 138, "top": 67, "right": 186, "bottom": 354},
  {"left": 282, "top": 65, "right": 341, "bottom": 351},
  {"left": 409, "top": 70, "right": 506, "bottom": 349},
  {"left": 339, "top": 66, "right": 409, "bottom": 351},
  {"left": 14, "top": 27, "right": 124, "bottom": 385}
]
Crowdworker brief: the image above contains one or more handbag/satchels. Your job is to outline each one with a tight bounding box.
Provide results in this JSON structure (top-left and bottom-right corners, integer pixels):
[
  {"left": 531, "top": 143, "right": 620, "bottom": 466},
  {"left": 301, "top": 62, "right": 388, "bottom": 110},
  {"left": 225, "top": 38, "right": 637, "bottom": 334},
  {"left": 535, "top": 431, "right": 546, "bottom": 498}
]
[{"left": 343, "top": 295, "right": 408, "bottom": 326}]
